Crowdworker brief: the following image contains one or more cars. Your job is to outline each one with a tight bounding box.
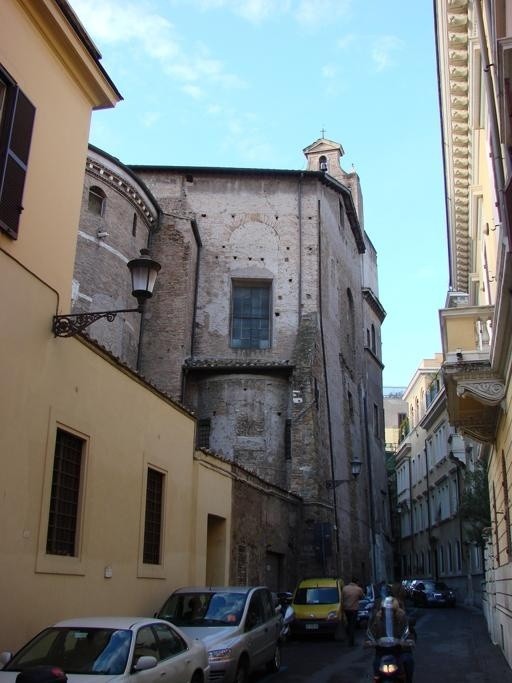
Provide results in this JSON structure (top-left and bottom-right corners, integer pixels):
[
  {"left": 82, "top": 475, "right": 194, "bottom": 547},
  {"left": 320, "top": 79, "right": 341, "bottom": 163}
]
[
  {"left": 1, "top": 617, "right": 211, "bottom": 682},
  {"left": 358, "top": 582, "right": 379, "bottom": 628},
  {"left": 400, "top": 579, "right": 456, "bottom": 608}
]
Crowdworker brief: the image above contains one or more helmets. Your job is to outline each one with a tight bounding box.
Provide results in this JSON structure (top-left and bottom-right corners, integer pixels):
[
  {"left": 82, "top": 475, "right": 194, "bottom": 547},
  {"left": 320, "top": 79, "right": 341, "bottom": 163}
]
[{"left": 380, "top": 596, "right": 399, "bottom": 618}]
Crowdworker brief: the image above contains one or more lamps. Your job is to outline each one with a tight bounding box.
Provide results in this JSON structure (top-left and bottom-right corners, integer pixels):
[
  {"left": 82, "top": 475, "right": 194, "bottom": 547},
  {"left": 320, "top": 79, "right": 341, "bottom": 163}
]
[
  {"left": 325, "top": 454, "right": 363, "bottom": 492},
  {"left": 50, "top": 245, "right": 163, "bottom": 338}
]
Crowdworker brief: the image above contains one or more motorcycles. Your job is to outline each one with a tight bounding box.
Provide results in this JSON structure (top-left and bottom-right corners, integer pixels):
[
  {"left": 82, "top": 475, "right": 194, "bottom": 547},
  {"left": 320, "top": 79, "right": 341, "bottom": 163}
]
[
  {"left": 277, "top": 591, "right": 293, "bottom": 639},
  {"left": 16, "top": 665, "right": 68, "bottom": 682},
  {"left": 364, "top": 616, "right": 419, "bottom": 683}
]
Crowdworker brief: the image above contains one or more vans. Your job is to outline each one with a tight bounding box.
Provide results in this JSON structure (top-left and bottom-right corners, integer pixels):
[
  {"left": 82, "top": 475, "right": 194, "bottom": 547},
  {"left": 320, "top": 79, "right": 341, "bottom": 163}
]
[
  {"left": 154, "top": 585, "right": 281, "bottom": 682},
  {"left": 289, "top": 577, "right": 345, "bottom": 640}
]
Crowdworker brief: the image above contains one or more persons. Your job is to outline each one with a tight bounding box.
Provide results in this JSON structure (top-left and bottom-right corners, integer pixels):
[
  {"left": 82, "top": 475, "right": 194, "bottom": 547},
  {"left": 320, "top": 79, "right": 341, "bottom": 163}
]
[
  {"left": 363, "top": 596, "right": 418, "bottom": 683},
  {"left": 340, "top": 575, "right": 365, "bottom": 648}
]
